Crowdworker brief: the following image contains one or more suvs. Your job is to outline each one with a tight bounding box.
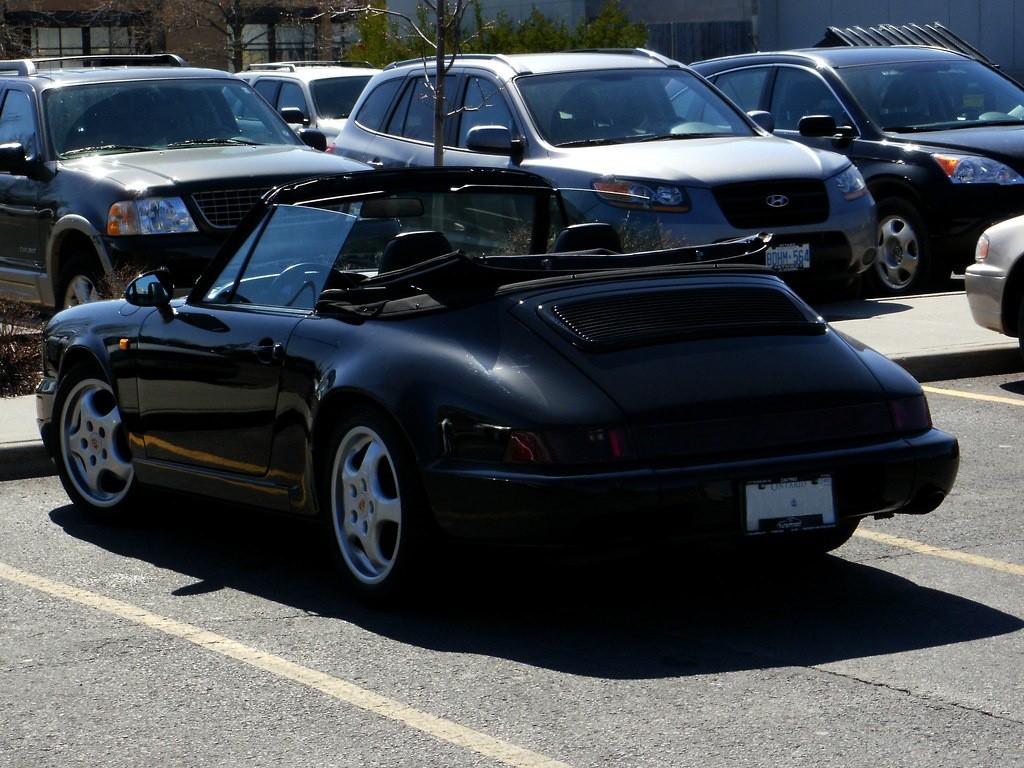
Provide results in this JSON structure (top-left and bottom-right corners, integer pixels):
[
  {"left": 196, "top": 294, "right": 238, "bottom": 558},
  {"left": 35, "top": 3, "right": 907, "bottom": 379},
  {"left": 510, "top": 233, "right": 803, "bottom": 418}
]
[
  {"left": 219, "top": 60, "right": 385, "bottom": 154},
  {"left": 321, "top": 48, "right": 880, "bottom": 290},
  {"left": 1, "top": 53, "right": 403, "bottom": 315}
]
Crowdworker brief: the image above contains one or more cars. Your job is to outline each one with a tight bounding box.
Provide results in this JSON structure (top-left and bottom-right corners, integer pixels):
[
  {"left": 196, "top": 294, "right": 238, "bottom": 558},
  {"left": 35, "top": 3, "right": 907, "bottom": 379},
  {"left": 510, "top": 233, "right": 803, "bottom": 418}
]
[
  {"left": 34, "top": 163, "right": 960, "bottom": 611},
  {"left": 637, "top": 44, "right": 1024, "bottom": 296},
  {"left": 965, "top": 212, "right": 1024, "bottom": 360}
]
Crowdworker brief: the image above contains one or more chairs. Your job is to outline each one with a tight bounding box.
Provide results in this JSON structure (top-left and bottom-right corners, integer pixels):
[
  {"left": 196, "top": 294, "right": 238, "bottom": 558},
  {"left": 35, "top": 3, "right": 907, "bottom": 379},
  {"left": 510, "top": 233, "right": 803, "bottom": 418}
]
[
  {"left": 380, "top": 229, "right": 454, "bottom": 273},
  {"left": 579, "top": 93, "right": 646, "bottom": 140},
  {"left": 778, "top": 81, "right": 831, "bottom": 125},
  {"left": 552, "top": 221, "right": 623, "bottom": 254},
  {"left": 875, "top": 71, "right": 930, "bottom": 125},
  {"left": 115, "top": 95, "right": 174, "bottom": 147}
]
